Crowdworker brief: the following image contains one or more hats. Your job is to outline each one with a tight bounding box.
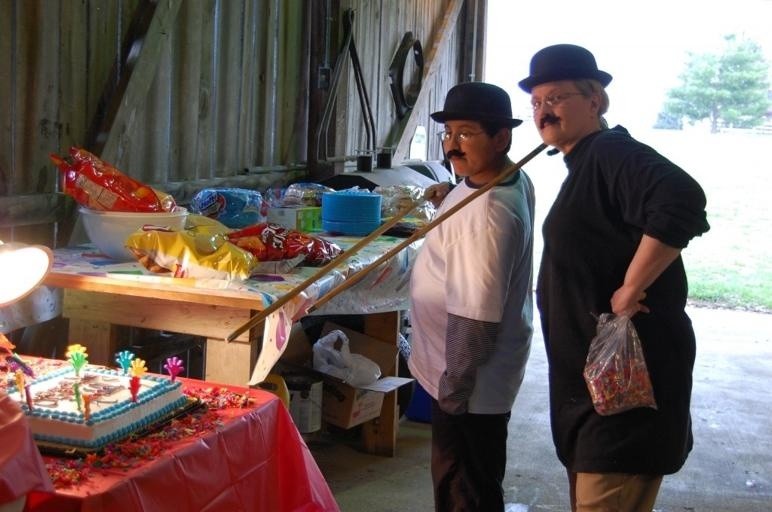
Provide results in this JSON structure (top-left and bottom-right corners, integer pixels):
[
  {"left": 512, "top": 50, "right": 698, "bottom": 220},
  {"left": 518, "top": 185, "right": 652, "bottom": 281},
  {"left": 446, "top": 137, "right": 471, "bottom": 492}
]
[
  {"left": 518, "top": 44, "right": 613, "bottom": 93},
  {"left": 430, "top": 83, "right": 522, "bottom": 127}
]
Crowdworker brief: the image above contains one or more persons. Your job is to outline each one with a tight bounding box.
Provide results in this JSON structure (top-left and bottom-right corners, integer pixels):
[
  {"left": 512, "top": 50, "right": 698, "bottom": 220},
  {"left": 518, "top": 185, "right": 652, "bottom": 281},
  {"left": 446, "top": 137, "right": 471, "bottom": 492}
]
[
  {"left": 518, "top": 43, "right": 710, "bottom": 512},
  {"left": 403, "top": 81, "right": 535, "bottom": 512}
]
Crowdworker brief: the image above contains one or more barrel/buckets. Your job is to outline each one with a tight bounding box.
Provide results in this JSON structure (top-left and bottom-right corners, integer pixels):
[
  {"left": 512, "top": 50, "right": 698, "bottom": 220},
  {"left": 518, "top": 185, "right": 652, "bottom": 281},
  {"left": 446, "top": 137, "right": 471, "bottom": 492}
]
[{"left": 281, "top": 369, "right": 324, "bottom": 434}]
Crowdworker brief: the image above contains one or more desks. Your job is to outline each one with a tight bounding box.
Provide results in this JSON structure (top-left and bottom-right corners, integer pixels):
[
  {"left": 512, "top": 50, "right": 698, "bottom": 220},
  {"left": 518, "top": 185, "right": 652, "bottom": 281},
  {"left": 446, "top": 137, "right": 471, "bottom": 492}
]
[
  {"left": 0, "top": 352, "right": 282, "bottom": 512},
  {"left": 43, "top": 233, "right": 420, "bottom": 457}
]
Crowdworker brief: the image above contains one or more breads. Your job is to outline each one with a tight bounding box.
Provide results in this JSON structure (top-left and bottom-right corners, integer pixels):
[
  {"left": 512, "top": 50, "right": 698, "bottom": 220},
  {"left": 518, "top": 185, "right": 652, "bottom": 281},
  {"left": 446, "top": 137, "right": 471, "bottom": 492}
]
[
  {"left": 374, "top": 186, "right": 433, "bottom": 221},
  {"left": 282, "top": 188, "right": 334, "bottom": 206}
]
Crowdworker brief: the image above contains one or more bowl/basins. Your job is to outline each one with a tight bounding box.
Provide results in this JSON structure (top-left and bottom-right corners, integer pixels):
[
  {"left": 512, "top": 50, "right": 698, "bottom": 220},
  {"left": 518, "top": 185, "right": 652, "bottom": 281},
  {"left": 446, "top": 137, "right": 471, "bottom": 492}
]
[{"left": 78, "top": 203, "right": 190, "bottom": 261}]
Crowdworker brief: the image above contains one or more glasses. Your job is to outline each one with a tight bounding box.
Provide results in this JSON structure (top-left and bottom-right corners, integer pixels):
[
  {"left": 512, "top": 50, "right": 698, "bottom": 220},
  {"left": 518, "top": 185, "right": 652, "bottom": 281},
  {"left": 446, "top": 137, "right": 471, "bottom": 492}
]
[
  {"left": 437, "top": 131, "right": 491, "bottom": 143},
  {"left": 531, "top": 92, "right": 585, "bottom": 110}
]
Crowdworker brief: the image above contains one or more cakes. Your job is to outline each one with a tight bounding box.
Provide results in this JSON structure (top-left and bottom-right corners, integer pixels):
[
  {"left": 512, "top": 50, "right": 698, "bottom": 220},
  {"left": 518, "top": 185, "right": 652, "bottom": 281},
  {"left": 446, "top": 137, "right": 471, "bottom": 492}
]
[{"left": 5, "top": 366, "right": 188, "bottom": 450}]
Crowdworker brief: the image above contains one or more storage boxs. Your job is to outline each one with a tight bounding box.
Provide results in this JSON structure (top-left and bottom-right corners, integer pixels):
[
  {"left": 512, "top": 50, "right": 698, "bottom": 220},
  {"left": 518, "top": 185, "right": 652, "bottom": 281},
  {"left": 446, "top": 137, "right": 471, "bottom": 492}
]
[{"left": 283, "top": 316, "right": 416, "bottom": 431}]
